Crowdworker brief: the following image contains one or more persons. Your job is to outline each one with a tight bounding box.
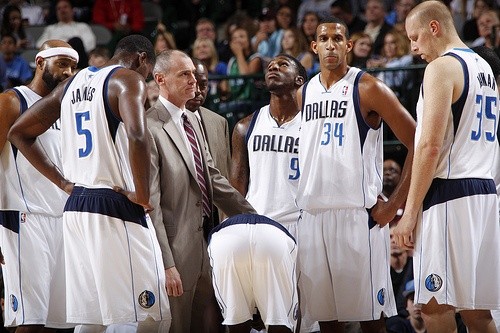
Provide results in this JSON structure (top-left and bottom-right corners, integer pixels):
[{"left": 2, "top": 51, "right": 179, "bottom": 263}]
[
  {"left": 7, "top": 34, "right": 171, "bottom": 333},
  {"left": 0, "top": 40, "right": 76, "bottom": 333},
  {"left": 186, "top": 58, "right": 234, "bottom": 230},
  {"left": 207, "top": 214, "right": 299, "bottom": 333},
  {"left": 0, "top": 0, "right": 500, "bottom": 133},
  {"left": 381, "top": 158, "right": 465, "bottom": 333},
  {"left": 394, "top": 0, "right": 500, "bottom": 333},
  {"left": 228, "top": 55, "right": 307, "bottom": 239},
  {"left": 296, "top": 18, "right": 417, "bottom": 333},
  {"left": 146, "top": 49, "right": 258, "bottom": 333}
]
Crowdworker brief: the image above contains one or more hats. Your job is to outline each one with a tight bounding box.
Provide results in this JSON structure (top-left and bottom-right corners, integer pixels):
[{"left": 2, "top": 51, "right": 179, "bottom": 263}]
[{"left": 402, "top": 279, "right": 414, "bottom": 299}]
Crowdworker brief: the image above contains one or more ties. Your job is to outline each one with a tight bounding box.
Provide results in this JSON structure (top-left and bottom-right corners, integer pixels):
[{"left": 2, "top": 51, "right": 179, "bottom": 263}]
[{"left": 181, "top": 112, "right": 212, "bottom": 219}]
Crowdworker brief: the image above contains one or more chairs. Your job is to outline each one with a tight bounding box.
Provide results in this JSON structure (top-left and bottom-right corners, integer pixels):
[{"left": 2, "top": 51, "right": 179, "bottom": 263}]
[
  {"left": 20, "top": 24, "right": 112, "bottom": 76},
  {"left": 137, "top": 2, "right": 162, "bottom": 36}
]
